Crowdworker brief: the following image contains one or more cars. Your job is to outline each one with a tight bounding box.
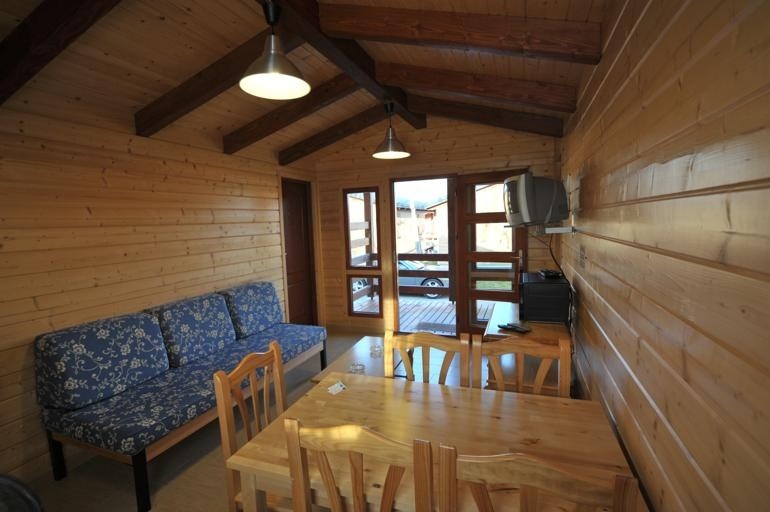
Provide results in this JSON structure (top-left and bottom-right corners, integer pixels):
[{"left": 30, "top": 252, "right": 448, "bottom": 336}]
[{"left": 349, "top": 253, "right": 449, "bottom": 299}]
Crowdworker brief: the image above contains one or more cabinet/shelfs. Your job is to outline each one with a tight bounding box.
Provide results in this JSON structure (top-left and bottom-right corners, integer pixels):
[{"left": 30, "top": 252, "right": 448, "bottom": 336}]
[{"left": 483, "top": 301, "right": 572, "bottom": 399}]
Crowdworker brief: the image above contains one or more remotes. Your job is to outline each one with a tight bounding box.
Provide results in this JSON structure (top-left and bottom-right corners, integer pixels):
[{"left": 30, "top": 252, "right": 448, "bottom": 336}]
[
  {"left": 507, "top": 323, "right": 531, "bottom": 332},
  {"left": 497, "top": 324, "right": 525, "bottom": 334}
]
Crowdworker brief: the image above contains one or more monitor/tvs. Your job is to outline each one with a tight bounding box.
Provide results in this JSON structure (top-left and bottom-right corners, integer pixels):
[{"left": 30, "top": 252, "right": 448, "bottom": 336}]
[{"left": 502, "top": 172, "right": 570, "bottom": 223}]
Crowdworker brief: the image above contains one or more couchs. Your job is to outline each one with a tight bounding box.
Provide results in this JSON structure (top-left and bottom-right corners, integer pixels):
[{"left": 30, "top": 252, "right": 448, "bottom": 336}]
[{"left": 33, "top": 281, "right": 326, "bottom": 511}]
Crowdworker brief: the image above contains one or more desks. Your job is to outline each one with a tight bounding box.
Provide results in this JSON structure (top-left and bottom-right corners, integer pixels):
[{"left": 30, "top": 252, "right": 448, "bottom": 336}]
[{"left": 226, "top": 371, "right": 651, "bottom": 512}]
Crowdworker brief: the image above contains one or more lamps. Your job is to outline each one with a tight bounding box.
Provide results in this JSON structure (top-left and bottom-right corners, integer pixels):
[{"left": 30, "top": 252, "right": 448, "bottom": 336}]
[
  {"left": 372, "top": 93, "right": 412, "bottom": 160},
  {"left": 238, "top": 0, "right": 313, "bottom": 102}
]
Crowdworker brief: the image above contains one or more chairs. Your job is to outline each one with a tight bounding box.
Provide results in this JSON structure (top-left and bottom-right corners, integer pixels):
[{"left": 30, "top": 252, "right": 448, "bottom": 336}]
[
  {"left": 286, "top": 418, "right": 433, "bottom": 510},
  {"left": 439, "top": 443, "right": 631, "bottom": 510},
  {"left": 382, "top": 329, "right": 470, "bottom": 387},
  {"left": 471, "top": 335, "right": 572, "bottom": 399},
  {"left": 214, "top": 341, "right": 293, "bottom": 510}
]
